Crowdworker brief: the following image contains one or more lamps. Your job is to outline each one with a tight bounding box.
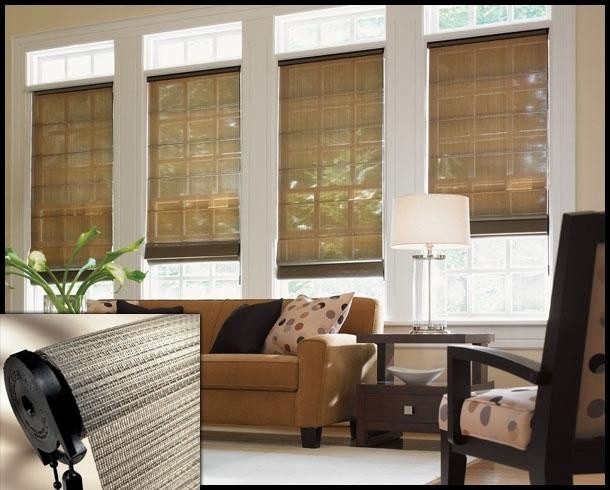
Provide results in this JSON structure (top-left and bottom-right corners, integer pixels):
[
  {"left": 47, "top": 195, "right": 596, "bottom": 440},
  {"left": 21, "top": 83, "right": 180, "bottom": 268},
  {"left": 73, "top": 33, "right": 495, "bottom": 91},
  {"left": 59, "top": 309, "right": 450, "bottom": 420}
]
[{"left": 390, "top": 194, "right": 471, "bottom": 334}]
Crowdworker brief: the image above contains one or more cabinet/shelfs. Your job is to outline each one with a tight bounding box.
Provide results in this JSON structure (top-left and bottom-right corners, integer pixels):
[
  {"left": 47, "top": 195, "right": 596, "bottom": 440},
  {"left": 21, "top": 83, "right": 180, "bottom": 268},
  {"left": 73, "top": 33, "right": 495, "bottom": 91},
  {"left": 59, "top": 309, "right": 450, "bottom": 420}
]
[{"left": 356, "top": 334, "right": 495, "bottom": 446}]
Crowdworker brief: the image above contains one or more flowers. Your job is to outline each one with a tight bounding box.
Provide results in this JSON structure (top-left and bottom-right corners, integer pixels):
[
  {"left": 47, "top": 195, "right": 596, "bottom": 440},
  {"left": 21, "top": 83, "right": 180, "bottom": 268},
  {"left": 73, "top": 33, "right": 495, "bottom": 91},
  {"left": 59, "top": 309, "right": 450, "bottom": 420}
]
[{"left": 5, "top": 226, "right": 150, "bottom": 314}]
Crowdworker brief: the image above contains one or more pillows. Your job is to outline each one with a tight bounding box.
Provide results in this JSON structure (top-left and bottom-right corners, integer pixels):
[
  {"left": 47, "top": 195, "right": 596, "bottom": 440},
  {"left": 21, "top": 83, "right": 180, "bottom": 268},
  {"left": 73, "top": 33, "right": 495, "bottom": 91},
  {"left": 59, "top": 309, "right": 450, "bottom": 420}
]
[
  {"left": 209, "top": 292, "right": 355, "bottom": 355},
  {"left": 87, "top": 299, "right": 183, "bottom": 314}
]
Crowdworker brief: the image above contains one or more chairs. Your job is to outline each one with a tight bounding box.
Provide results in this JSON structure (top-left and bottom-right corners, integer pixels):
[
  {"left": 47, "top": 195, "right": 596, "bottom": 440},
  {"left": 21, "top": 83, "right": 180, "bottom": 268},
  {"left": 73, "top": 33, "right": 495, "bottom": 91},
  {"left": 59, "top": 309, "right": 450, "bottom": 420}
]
[{"left": 437, "top": 212, "right": 606, "bottom": 485}]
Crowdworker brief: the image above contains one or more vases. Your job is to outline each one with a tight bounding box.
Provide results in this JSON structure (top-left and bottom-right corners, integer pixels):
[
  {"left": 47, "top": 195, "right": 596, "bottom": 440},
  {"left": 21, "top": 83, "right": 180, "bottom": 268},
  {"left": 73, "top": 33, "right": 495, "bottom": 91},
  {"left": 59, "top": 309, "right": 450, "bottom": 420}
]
[{"left": 44, "top": 295, "right": 86, "bottom": 313}]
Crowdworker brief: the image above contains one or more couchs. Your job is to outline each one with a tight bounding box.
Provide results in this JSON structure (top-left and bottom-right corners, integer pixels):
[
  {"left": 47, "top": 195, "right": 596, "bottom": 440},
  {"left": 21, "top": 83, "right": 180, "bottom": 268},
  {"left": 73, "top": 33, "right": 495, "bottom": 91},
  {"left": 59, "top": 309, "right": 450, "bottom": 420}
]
[{"left": 98, "top": 297, "right": 383, "bottom": 448}]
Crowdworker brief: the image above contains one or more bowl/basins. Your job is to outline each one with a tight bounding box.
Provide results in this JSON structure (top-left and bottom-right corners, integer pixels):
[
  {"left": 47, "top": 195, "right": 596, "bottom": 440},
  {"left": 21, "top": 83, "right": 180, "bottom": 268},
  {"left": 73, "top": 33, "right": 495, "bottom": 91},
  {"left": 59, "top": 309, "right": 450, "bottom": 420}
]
[{"left": 386, "top": 366, "right": 446, "bottom": 385}]
